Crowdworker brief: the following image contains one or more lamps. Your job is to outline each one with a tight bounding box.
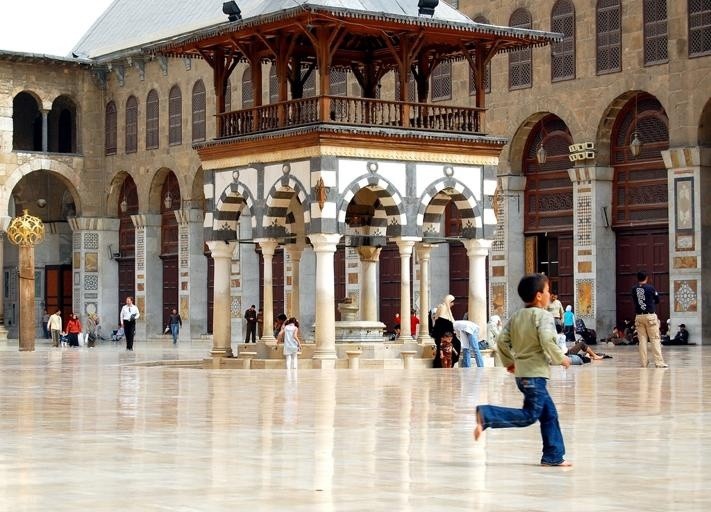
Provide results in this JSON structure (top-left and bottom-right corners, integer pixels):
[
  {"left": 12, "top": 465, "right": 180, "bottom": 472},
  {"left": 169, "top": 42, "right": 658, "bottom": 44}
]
[
  {"left": 164, "top": 171, "right": 175, "bottom": 210},
  {"left": 631, "top": 91, "right": 645, "bottom": 159},
  {"left": 538, "top": 117, "right": 547, "bottom": 165},
  {"left": 418, "top": 0, "right": 440, "bottom": 17},
  {"left": 220, "top": 0, "right": 243, "bottom": 21},
  {"left": 120, "top": 176, "right": 129, "bottom": 213}
]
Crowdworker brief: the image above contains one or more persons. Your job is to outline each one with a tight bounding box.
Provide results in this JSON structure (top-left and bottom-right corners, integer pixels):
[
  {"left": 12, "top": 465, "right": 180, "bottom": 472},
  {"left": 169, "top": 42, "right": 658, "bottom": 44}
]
[
  {"left": 44, "top": 311, "right": 97, "bottom": 348},
  {"left": 428, "top": 295, "right": 483, "bottom": 368},
  {"left": 473, "top": 272, "right": 577, "bottom": 468},
  {"left": 256, "top": 309, "right": 264, "bottom": 340},
  {"left": 120, "top": 296, "right": 139, "bottom": 351},
  {"left": 547, "top": 294, "right": 604, "bottom": 365},
  {"left": 278, "top": 317, "right": 301, "bottom": 369},
  {"left": 244, "top": 305, "right": 256, "bottom": 342},
  {"left": 606, "top": 319, "right": 638, "bottom": 346},
  {"left": 167, "top": 309, "right": 182, "bottom": 343},
  {"left": 631, "top": 271, "right": 669, "bottom": 367},
  {"left": 274, "top": 313, "right": 287, "bottom": 341},
  {"left": 661, "top": 324, "right": 688, "bottom": 345},
  {"left": 487, "top": 295, "right": 503, "bottom": 368},
  {"left": 394, "top": 309, "right": 419, "bottom": 340}
]
